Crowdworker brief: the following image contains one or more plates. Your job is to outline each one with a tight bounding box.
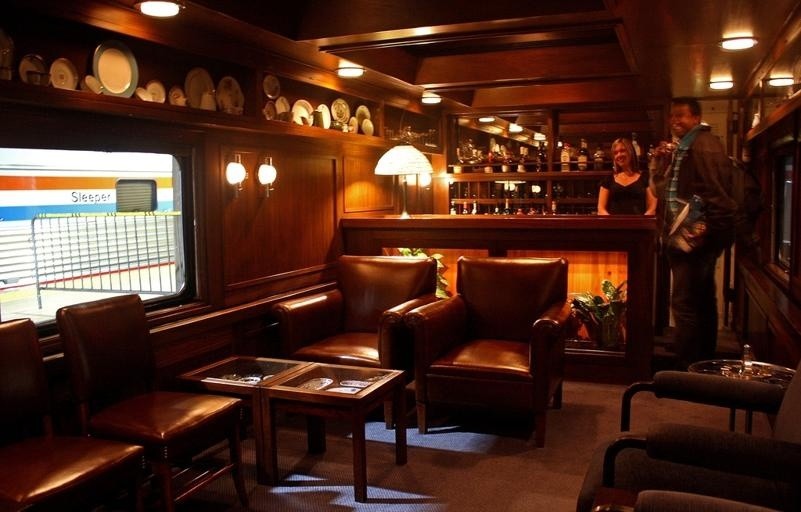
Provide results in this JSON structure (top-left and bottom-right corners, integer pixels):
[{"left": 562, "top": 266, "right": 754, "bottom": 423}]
[
  {"left": 263, "top": 75, "right": 374, "bottom": 135},
  {"left": 222, "top": 374, "right": 373, "bottom": 395},
  {"left": 19, "top": 41, "right": 244, "bottom": 116}
]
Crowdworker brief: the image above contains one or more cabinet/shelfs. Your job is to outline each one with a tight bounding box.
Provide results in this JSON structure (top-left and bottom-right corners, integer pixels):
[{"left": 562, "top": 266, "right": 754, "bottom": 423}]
[
  {"left": 447, "top": 162, "right": 549, "bottom": 218},
  {"left": 553, "top": 160, "right": 654, "bottom": 217},
  {"left": 734, "top": 123, "right": 796, "bottom": 365}
]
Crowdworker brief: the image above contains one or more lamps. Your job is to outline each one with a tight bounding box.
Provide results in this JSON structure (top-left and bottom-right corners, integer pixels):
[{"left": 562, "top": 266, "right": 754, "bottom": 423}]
[
  {"left": 255, "top": 157, "right": 276, "bottom": 197},
  {"left": 373, "top": 142, "right": 434, "bottom": 219},
  {"left": 227, "top": 154, "right": 249, "bottom": 193}
]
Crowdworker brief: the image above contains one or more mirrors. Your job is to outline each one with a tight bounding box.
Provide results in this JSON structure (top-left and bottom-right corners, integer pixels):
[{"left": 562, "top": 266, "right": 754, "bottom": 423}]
[
  {"left": 553, "top": 106, "right": 660, "bottom": 167},
  {"left": 447, "top": 111, "right": 554, "bottom": 167}
]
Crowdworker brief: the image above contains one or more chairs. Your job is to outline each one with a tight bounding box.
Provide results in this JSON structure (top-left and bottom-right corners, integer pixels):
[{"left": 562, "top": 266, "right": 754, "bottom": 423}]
[
  {"left": 576, "top": 351, "right": 801, "bottom": 511},
  {"left": 57, "top": 294, "right": 251, "bottom": 510},
  {"left": 0, "top": 317, "right": 146, "bottom": 512},
  {"left": 405, "top": 256, "right": 565, "bottom": 447},
  {"left": 270, "top": 255, "right": 437, "bottom": 430}
]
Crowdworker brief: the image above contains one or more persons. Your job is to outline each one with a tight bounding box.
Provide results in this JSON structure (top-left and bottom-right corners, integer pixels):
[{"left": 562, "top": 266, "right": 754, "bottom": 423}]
[
  {"left": 596, "top": 137, "right": 658, "bottom": 219},
  {"left": 650, "top": 96, "right": 739, "bottom": 364}
]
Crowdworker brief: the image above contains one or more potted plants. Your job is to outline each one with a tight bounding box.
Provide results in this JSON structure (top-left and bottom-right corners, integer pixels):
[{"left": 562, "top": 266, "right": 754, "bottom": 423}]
[{"left": 575, "top": 280, "right": 628, "bottom": 346}]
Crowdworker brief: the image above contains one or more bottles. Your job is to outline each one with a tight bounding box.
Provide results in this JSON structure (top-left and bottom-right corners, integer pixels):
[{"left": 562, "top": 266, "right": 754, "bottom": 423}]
[
  {"left": 449, "top": 198, "right": 556, "bottom": 215},
  {"left": 743, "top": 345, "right": 753, "bottom": 375},
  {"left": 453, "top": 132, "right": 654, "bottom": 173}
]
